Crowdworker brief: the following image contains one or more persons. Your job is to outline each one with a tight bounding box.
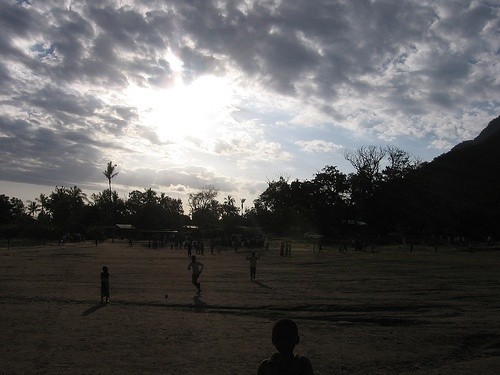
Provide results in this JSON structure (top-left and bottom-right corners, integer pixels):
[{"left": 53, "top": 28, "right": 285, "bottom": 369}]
[
  {"left": 246, "top": 252, "right": 260, "bottom": 281},
  {"left": 187, "top": 255, "right": 204, "bottom": 294},
  {"left": 257, "top": 319, "right": 315, "bottom": 375},
  {"left": 100, "top": 265, "right": 110, "bottom": 303},
  {"left": 58, "top": 230, "right": 500, "bottom": 259}
]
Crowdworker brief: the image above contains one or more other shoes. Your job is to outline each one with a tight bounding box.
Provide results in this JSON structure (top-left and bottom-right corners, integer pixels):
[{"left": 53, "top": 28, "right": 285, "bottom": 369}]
[
  {"left": 250, "top": 277, "right": 256, "bottom": 280},
  {"left": 100, "top": 299, "right": 109, "bottom": 304},
  {"left": 197, "top": 289, "right": 202, "bottom": 294}
]
